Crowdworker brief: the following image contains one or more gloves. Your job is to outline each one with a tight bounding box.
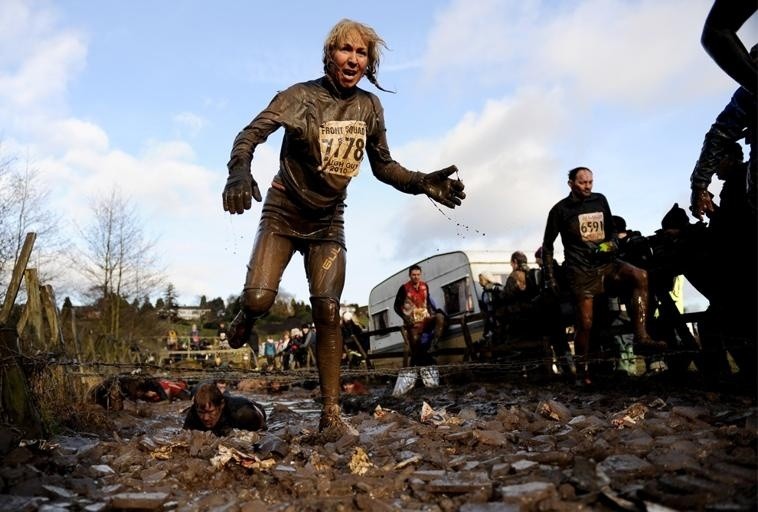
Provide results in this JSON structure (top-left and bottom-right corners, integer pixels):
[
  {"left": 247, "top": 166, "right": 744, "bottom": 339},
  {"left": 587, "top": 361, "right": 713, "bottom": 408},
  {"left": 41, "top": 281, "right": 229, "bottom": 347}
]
[
  {"left": 420, "top": 165, "right": 466, "bottom": 209},
  {"left": 221, "top": 167, "right": 262, "bottom": 214}
]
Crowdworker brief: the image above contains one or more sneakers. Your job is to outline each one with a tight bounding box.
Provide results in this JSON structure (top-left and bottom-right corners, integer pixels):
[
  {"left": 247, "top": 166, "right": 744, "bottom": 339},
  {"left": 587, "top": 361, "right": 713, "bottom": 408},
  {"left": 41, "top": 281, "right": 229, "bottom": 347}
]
[
  {"left": 318, "top": 412, "right": 361, "bottom": 442},
  {"left": 227, "top": 310, "right": 256, "bottom": 348}
]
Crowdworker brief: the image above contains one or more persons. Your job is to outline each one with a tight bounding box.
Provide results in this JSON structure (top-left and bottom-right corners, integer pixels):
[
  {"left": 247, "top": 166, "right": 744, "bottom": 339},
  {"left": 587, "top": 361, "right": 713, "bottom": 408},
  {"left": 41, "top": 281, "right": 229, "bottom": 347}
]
[
  {"left": 700, "top": 0, "right": 758, "bottom": 99},
  {"left": 611, "top": 215, "right": 658, "bottom": 319},
  {"left": 532, "top": 247, "right": 572, "bottom": 359},
  {"left": 690, "top": 43, "right": 758, "bottom": 221},
  {"left": 268, "top": 377, "right": 283, "bottom": 394},
  {"left": 542, "top": 167, "right": 669, "bottom": 392},
  {"left": 688, "top": 143, "right": 758, "bottom": 393},
  {"left": 222, "top": 18, "right": 466, "bottom": 437},
  {"left": 661, "top": 202, "right": 735, "bottom": 384},
  {"left": 340, "top": 377, "right": 366, "bottom": 394},
  {"left": 478, "top": 270, "right": 505, "bottom": 347},
  {"left": 499, "top": 251, "right": 543, "bottom": 362},
  {"left": 184, "top": 381, "right": 267, "bottom": 436},
  {"left": 215, "top": 380, "right": 230, "bottom": 396},
  {"left": 264, "top": 322, "right": 317, "bottom": 371},
  {"left": 164, "top": 322, "right": 229, "bottom": 368},
  {"left": 393, "top": 264, "right": 445, "bottom": 367}
]
